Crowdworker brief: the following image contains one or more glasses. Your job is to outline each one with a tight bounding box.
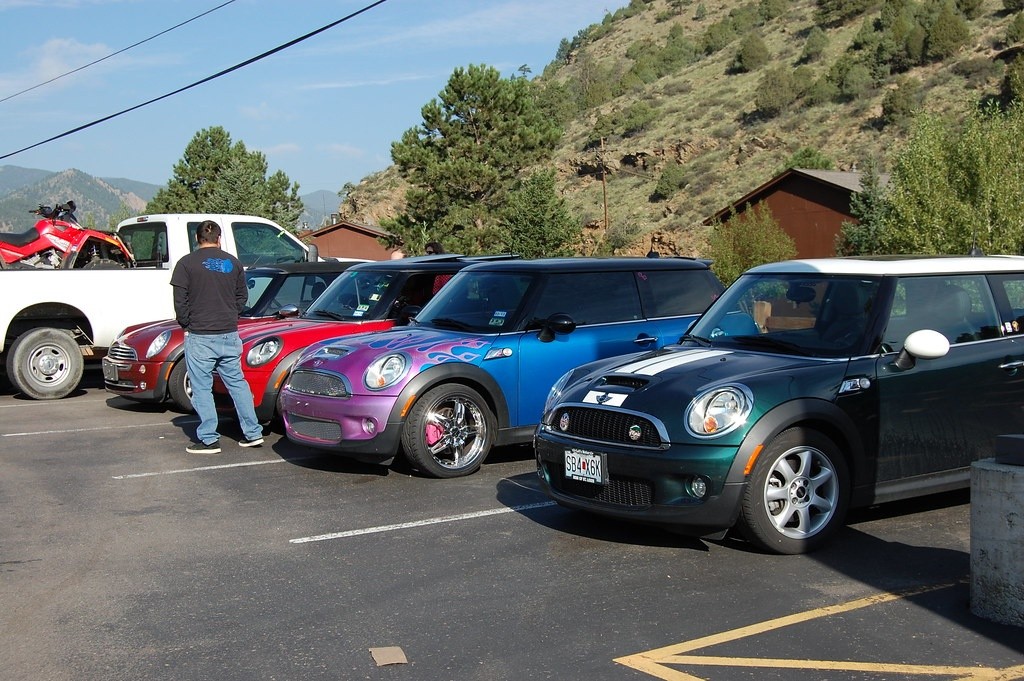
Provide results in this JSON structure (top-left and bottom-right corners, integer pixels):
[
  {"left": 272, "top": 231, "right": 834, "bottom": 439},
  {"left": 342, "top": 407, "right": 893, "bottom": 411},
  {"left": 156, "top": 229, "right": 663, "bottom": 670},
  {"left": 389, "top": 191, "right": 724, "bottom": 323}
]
[{"left": 426, "top": 251, "right": 435, "bottom": 255}]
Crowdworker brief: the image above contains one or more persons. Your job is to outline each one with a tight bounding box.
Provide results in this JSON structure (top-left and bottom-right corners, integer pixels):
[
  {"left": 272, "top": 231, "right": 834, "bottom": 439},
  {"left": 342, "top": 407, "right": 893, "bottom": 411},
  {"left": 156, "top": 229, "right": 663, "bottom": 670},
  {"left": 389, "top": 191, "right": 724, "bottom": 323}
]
[{"left": 170, "top": 219, "right": 265, "bottom": 454}]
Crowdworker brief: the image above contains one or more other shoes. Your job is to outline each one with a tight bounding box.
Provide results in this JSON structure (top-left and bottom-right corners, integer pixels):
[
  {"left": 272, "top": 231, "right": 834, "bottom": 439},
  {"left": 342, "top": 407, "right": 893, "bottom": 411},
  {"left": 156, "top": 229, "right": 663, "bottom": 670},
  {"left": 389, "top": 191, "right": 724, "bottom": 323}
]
[
  {"left": 239, "top": 437, "right": 264, "bottom": 447},
  {"left": 186, "top": 439, "right": 221, "bottom": 453}
]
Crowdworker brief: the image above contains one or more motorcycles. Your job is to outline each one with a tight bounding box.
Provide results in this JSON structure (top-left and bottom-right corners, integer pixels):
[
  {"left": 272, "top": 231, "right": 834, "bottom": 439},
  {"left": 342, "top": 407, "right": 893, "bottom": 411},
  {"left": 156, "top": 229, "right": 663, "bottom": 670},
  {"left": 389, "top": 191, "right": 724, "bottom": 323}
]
[{"left": 0, "top": 200, "right": 136, "bottom": 271}]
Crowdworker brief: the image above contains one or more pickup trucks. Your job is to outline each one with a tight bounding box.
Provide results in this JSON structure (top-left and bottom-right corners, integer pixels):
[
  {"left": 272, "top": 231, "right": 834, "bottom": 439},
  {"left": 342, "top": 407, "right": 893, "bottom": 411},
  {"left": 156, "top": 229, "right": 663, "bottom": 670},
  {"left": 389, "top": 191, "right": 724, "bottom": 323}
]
[{"left": 0, "top": 212, "right": 378, "bottom": 402}]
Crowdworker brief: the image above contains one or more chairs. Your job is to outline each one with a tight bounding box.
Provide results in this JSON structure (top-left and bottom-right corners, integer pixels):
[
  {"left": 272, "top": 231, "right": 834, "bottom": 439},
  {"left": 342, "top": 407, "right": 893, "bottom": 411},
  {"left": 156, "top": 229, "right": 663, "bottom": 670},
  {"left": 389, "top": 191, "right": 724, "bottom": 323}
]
[
  {"left": 909, "top": 284, "right": 980, "bottom": 346},
  {"left": 821, "top": 283, "right": 879, "bottom": 345},
  {"left": 299, "top": 282, "right": 326, "bottom": 307}
]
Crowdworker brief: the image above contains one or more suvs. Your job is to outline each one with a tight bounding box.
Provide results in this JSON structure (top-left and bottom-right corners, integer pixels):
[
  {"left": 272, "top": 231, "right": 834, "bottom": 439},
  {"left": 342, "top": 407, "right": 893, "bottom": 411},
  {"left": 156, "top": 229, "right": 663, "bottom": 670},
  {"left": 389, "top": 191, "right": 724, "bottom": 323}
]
[
  {"left": 528, "top": 253, "right": 1023, "bottom": 557},
  {"left": 278, "top": 251, "right": 763, "bottom": 478},
  {"left": 101, "top": 259, "right": 380, "bottom": 415},
  {"left": 211, "top": 252, "right": 520, "bottom": 430}
]
